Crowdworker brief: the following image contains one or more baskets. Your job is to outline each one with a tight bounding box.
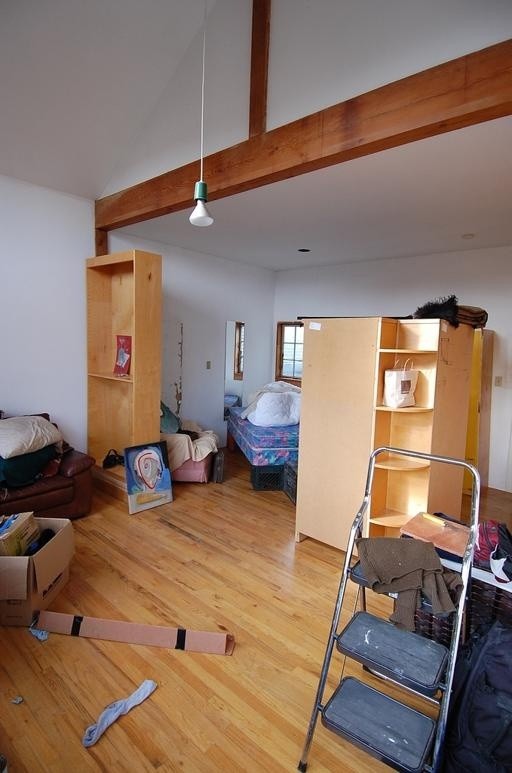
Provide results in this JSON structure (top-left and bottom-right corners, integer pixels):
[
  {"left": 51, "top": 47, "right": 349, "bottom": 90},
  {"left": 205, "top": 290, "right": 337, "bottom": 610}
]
[
  {"left": 284, "top": 462, "right": 297, "bottom": 505},
  {"left": 252, "top": 466, "right": 284, "bottom": 491}
]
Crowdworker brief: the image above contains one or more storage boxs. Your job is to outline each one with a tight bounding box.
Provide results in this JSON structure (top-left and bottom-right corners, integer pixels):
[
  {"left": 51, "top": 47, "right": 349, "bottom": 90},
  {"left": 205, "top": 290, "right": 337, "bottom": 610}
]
[
  {"left": 0, "top": 518, "right": 76, "bottom": 627},
  {"left": 0, "top": 512, "right": 41, "bottom": 556}
]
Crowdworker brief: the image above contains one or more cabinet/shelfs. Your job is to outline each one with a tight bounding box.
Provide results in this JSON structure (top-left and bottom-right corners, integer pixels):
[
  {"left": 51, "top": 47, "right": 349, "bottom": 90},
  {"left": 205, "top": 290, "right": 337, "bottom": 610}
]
[
  {"left": 295, "top": 318, "right": 473, "bottom": 558},
  {"left": 460, "top": 322, "right": 495, "bottom": 502},
  {"left": 86, "top": 249, "right": 163, "bottom": 506}
]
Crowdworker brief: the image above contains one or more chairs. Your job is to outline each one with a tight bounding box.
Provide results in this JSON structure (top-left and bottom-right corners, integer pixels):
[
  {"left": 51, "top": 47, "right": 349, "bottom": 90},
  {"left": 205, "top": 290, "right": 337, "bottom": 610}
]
[{"left": 160, "top": 401, "right": 219, "bottom": 483}]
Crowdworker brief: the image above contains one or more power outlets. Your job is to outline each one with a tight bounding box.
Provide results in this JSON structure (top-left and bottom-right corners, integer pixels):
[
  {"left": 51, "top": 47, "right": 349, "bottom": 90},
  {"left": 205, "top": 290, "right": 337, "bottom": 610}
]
[{"left": 495, "top": 376, "right": 502, "bottom": 386}]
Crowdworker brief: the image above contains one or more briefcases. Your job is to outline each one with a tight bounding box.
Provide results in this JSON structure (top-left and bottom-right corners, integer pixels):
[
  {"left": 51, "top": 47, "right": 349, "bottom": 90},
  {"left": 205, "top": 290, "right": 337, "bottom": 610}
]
[{"left": 213, "top": 450, "right": 225, "bottom": 483}]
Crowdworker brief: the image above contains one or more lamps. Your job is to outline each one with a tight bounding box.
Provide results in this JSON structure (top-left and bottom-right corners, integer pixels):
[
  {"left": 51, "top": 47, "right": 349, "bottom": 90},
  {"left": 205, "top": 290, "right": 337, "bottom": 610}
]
[{"left": 189, "top": 0, "right": 214, "bottom": 227}]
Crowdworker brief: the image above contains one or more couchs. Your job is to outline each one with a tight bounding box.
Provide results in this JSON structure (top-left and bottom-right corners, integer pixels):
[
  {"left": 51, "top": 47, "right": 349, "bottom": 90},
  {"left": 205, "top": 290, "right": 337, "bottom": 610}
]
[{"left": 0, "top": 413, "right": 97, "bottom": 520}]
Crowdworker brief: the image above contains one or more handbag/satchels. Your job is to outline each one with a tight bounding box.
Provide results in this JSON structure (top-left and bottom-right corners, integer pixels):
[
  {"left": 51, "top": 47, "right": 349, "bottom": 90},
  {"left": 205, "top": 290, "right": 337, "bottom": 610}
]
[
  {"left": 472, "top": 519, "right": 512, "bottom": 577},
  {"left": 446, "top": 620, "right": 511, "bottom": 773},
  {"left": 381, "top": 358, "right": 419, "bottom": 408}
]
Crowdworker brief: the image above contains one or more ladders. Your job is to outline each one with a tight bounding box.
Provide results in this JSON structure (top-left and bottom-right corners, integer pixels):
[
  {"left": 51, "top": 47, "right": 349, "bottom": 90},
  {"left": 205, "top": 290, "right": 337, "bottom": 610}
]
[{"left": 297, "top": 447, "right": 481, "bottom": 773}]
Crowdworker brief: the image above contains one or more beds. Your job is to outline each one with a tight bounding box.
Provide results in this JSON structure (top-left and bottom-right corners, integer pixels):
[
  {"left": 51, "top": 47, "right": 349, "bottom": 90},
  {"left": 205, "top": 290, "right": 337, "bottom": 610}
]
[
  {"left": 224, "top": 395, "right": 242, "bottom": 421},
  {"left": 227, "top": 406, "right": 300, "bottom": 491}
]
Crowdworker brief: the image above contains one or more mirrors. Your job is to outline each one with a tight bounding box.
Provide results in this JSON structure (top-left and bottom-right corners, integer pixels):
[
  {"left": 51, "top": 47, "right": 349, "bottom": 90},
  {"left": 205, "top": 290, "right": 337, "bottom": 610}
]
[{"left": 224, "top": 320, "right": 245, "bottom": 422}]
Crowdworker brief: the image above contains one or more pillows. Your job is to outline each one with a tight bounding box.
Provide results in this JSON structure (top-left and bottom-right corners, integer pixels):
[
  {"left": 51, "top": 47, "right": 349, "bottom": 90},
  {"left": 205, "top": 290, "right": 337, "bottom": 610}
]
[{"left": 0, "top": 415, "right": 65, "bottom": 460}]
